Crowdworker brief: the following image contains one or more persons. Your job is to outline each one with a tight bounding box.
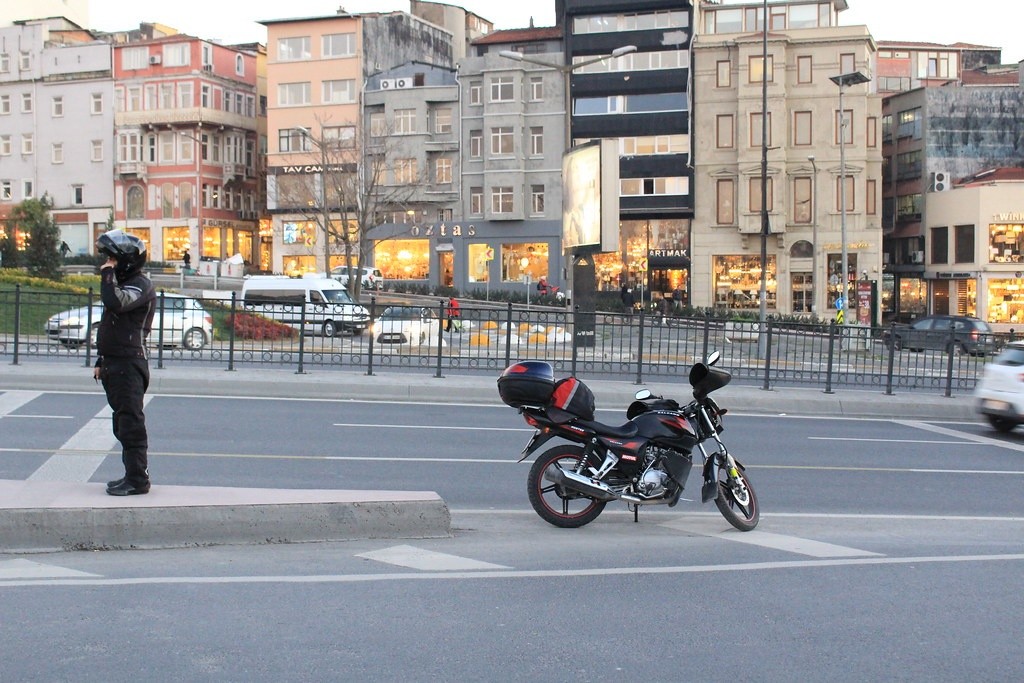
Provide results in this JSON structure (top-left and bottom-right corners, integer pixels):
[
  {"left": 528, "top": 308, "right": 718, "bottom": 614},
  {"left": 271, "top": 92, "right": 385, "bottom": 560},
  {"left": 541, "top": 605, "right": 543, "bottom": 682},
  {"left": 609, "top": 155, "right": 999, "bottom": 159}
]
[
  {"left": 672, "top": 284, "right": 683, "bottom": 310},
  {"left": 183, "top": 250, "right": 190, "bottom": 269},
  {"left": 443, "top": 297, "right": 459, "bottom": 333},
  {"left": 537, "top": 276, "right": 553, "bottom": 305},
  {"left": 621, "top": 283, "right": 627, "bottom": 303},
  {"left": 91, "top": 230, "right": 157, "bottom": 496},
  {"left": 60, "top": 240, "right": 72, "bottom": 259},
  {"left": 623, "top": 289, "right": 633, "bottom": 322}
]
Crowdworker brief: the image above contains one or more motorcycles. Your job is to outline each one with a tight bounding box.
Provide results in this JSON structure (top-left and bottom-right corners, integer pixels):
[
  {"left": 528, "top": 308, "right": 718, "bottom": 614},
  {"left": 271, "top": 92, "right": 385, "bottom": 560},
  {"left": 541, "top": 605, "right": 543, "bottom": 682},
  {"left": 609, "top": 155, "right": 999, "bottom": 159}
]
[{"left": 496, "top": 349, "right": 760, "bottom": 534}]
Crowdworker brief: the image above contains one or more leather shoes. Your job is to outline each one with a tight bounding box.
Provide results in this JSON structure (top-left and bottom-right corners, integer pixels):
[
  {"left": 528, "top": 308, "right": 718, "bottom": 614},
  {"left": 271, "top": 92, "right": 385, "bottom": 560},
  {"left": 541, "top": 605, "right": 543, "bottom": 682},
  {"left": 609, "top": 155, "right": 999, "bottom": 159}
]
[{"left": 106, "top": 477, "right": 150, "bottom": 496}]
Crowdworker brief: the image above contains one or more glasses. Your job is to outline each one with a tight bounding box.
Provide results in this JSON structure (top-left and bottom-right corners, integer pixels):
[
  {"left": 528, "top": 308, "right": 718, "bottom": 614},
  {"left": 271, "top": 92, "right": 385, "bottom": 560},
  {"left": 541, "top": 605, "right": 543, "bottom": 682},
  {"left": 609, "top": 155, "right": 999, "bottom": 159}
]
[{"left": 104, "top": 258, "right": 116, "bottom": 262}]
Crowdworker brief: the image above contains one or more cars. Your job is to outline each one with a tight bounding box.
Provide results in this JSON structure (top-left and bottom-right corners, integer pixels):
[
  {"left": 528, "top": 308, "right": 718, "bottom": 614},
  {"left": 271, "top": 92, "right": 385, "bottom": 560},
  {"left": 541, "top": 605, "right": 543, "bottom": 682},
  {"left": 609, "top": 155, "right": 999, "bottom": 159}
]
[
  {"left": 883, "top": 313, "right": 998, "bottom": 357},
  {"left": 373, "top": 305, "right": 442, "bottom": 346},
  {"left": 973, "top": 339, "right": 1024, "bottom": 434},
  {"left": 320, "top": 264, "right": 384, "bottom": 290},
  {"left": 43, "top": 291, "right": 214, "bottom": 351}
]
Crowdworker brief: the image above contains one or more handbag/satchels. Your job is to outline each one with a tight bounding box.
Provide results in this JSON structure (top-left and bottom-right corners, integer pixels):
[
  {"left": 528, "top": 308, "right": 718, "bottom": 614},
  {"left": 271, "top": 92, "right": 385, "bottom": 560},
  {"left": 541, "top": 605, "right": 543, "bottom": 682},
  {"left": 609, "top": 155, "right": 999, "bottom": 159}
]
[{"left": 537, "top": 281, "right": 546, "bottom": 290}]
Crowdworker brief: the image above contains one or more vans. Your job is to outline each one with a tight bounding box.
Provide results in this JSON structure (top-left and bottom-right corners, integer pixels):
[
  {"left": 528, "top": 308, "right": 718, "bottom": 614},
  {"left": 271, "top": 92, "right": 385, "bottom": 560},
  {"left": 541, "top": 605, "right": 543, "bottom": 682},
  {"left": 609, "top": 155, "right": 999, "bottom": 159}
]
[{"left": 242, "top": 277, "right": 370, "bottom": 338}]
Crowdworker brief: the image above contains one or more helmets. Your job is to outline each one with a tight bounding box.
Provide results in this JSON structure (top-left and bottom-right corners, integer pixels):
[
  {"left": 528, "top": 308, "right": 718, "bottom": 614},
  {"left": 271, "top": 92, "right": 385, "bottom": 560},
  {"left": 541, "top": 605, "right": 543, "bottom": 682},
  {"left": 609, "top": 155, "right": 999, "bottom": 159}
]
[{"left": 95, "top": 228, "right": 147, "bottom": 280}]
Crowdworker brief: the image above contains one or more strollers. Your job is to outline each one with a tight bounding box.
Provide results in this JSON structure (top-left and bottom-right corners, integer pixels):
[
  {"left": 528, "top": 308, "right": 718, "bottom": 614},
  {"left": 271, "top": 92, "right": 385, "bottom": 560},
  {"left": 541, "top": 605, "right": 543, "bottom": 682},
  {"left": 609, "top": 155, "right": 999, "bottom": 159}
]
[{"left": 552, "top": 286, "right": 566, "bottom": 306}]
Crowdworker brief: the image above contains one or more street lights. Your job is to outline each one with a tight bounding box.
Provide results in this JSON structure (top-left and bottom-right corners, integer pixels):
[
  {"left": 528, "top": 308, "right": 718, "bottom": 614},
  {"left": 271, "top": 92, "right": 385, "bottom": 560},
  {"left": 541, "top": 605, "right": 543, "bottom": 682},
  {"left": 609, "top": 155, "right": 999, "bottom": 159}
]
[
  {"left": 497, "top": 44, "right": 637, "bottom": 333},
  {"left": 296, "top": 126, "right": 332, "bottom": 278},
  {"left": 807, "top": 156, "right": 816, "bottom": 317},
  {"left": 829, "top": 71, "right": 871, "bottom": 350},
  {"left": 180, "top": 132, "right": 202, "bottom": 272}
]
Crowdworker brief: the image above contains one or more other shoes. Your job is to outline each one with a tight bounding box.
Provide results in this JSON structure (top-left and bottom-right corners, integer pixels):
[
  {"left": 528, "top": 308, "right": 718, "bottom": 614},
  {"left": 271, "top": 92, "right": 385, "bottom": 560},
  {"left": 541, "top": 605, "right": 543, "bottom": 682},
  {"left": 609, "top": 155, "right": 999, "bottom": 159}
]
[
  {"left": 444, "top": 329, "right": 449, "bottom": 332},
  {"left": 455, "top": 330, "right": 458, "bottom": 332}
]
